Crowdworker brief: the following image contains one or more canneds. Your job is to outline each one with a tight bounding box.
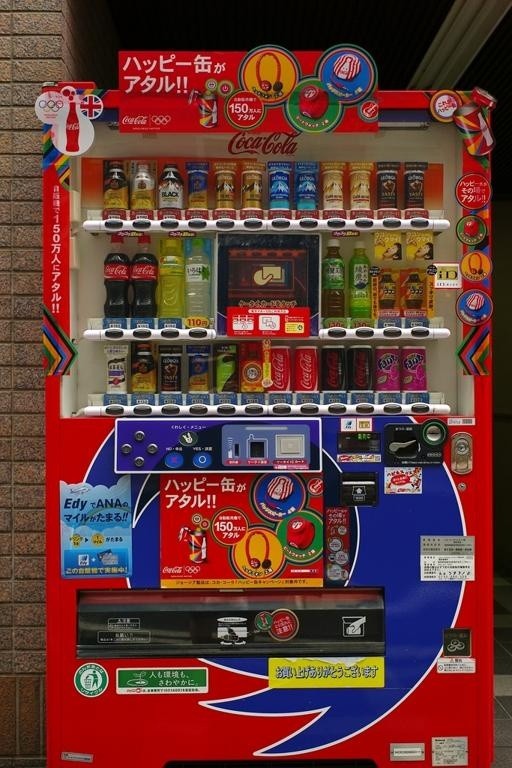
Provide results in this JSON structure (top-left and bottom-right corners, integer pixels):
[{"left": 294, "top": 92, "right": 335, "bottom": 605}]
[
  {"left": 269, "top": 346, "right": 291, "bottom": 391},
  {"left": 401, "top": 347, "right": 426, "bottom": 390},
  {"left": 321, "top": 344, "right": 345, "bottom": 389},
  {"left": 374, "top": 346, "right": 401, "bottom": 390},
  {"left": 295, "top": 345, "right": 320, "bottom": 391},
  {"left": 347, "top": 344, "right": 371, "bottom": 390}
]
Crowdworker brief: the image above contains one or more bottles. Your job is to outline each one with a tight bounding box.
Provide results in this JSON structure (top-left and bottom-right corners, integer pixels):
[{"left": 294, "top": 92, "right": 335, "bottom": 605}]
[
  {"left": 337, "top": 56, "right": 352, "bottom": 80},
  {"left": 379, "top": 265, "right": 425, "bottom": 310},
  {"left": 189, "top": 525, "right": 207, "bottom": 563},
  {"left": 64, "top": 102, "right": 81, "bottom": 155},
  {"left": 103, "top": 233, "right": 211, "bottom": 320},
  {"left": 322, "top": 238, "right": 345, "bottom": 318},
  {"left": 348, "top": 240, "right": 372, "bottom": 318},
  {"left": 103, "top": 159, "right": 184, "bottom": 210},
  {"left": 132, "top": 343, "right": 156, "bottom": 393},
  {"left": 271, "top": 478, "right": 286, "bottom": 500}
]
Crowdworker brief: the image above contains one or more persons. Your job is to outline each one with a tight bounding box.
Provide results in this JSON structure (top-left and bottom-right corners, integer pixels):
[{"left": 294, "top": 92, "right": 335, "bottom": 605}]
[
  {"left": 382, "top": 241, "right": 402, "bottom": 261},
  {"left": 414, "top": 242, "right": 433, "bottom": 261}
]
